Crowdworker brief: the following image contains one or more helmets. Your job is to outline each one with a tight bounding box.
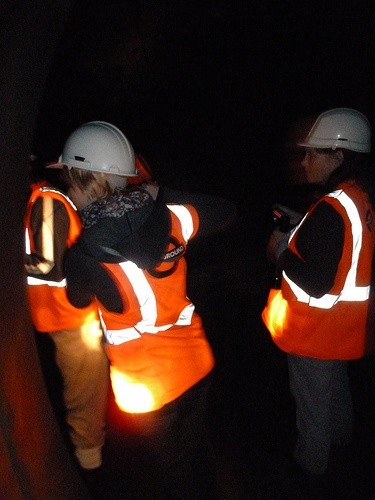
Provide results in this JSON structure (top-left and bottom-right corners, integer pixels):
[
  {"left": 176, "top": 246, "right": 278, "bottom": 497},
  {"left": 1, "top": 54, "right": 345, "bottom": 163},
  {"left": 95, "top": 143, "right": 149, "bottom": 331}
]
[
  {"left": 47, "top": 120, "right": 140, "bottom": 178},
  {"left": 296, "top": 107, "right": 375, "bottom": 155}
]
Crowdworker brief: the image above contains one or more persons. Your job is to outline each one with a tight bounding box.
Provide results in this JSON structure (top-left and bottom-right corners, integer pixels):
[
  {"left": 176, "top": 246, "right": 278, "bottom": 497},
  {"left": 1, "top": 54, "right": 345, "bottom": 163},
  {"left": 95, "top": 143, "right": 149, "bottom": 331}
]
[
  {"left": 26, "top": 136, "right": 117, "bottom": 496},
  {"left": 263, "top": 107, "right": 374, "bottom": 488},
  {"left": 30, "top": 119, "right": 216, "bottom": 500}
]
